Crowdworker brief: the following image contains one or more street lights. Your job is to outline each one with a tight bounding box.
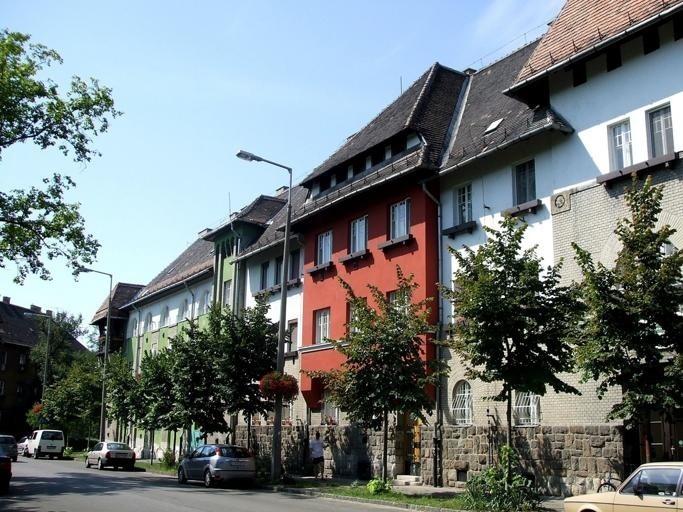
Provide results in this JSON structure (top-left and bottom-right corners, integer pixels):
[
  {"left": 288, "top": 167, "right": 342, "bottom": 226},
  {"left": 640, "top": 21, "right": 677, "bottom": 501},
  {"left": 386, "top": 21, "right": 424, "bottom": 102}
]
[
  {"left": 80, "top": 267, "right": 112, "bottom": 441},
  {"left": 22, "top": 312, "right": 53, "bottom": 428},
  {"left": 235, "top": 149, "right": 293, "bottom": 485}
]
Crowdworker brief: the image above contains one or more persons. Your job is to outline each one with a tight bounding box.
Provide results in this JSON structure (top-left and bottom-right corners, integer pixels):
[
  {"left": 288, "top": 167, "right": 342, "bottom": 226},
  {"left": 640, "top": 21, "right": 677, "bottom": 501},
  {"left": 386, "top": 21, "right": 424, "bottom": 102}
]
[{"left": 309, "top": 432, "right": 329, "bottom": 481}]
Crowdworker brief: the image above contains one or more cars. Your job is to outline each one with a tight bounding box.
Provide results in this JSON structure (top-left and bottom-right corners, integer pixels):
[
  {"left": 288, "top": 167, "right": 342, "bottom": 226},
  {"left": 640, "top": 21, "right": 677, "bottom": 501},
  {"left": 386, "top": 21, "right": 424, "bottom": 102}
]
[
  {"left": 15, "top": 436, "right": 30, "bottom": 456},
  {"left": 84, "top": 442, "right": 135, "bottom": 470},
  {"left": 177, "top": 443, "right": 256, "bottom": 488},
  {"left": 0, "top": 434, "right": 18, "bottom": 462},
  {"left": 563, "top": 459, "right": 683, "bottom": 512}
]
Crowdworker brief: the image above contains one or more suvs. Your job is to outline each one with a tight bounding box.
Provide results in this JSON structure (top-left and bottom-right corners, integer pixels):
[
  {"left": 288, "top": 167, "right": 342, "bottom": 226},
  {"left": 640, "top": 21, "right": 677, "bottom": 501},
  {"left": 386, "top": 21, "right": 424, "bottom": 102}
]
[{"left": 23, "top": 429, "right": 65, "bottom": 459}]
